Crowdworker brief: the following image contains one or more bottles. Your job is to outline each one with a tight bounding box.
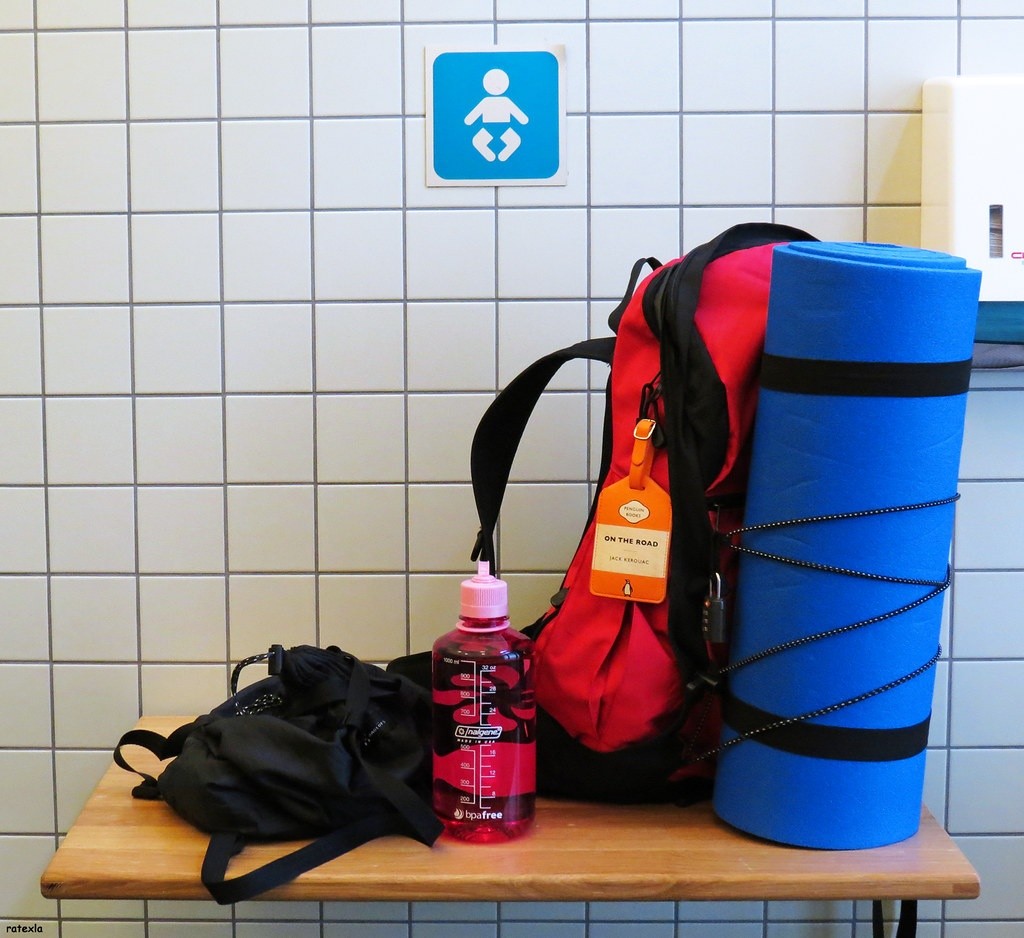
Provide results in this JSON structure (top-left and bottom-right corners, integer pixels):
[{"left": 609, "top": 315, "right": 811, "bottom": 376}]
[{"left": 430, "top": 563, "right": 538, "bottom": 847}]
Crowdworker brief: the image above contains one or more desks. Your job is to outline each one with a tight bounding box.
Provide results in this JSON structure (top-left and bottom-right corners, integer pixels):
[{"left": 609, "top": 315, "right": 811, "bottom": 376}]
[{"left": 41, "top": 710, "right": 980, "bottom": 938}]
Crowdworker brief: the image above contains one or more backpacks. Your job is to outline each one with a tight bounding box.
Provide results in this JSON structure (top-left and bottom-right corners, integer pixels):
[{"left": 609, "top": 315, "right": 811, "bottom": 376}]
[
  {"left": 470, "top": 223, "right": 820, "bottom": 807},
  {"left": 113, "top": 643, "right": 445, "bottom": 905}
]
